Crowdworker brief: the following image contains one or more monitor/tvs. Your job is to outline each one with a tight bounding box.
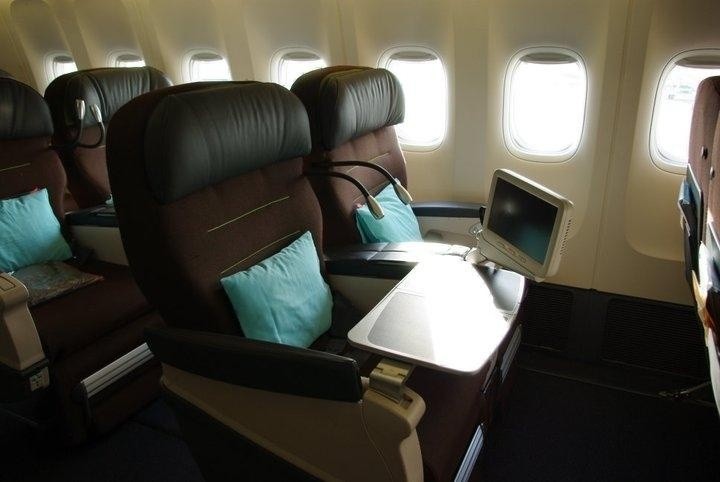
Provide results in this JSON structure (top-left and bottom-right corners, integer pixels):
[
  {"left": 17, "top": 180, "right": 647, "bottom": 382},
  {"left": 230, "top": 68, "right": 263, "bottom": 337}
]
[{"left": 482, "top": 168, "right": 574, "bottom": 279}]
